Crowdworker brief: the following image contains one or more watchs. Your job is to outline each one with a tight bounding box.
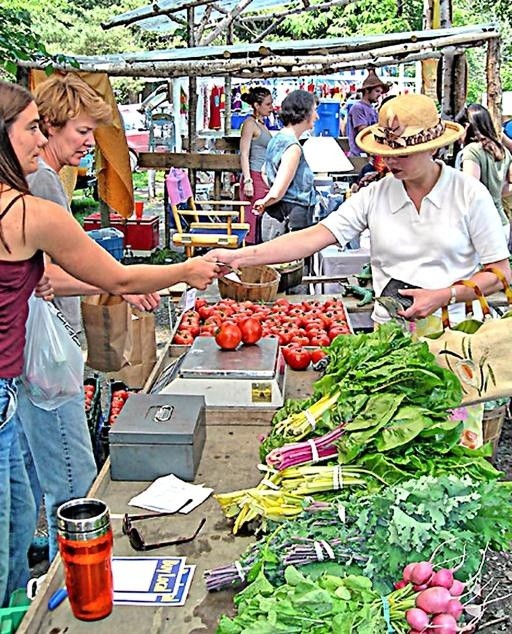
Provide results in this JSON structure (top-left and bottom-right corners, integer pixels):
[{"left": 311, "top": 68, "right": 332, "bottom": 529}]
[{"left": 449, "top": 286, "right": 456, "bottom": 305}]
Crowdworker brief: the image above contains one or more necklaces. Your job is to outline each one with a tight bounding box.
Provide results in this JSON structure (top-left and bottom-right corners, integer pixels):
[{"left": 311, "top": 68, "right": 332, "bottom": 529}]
[{"left": 252, "top": 115, "right": 265, "bottom": 125}]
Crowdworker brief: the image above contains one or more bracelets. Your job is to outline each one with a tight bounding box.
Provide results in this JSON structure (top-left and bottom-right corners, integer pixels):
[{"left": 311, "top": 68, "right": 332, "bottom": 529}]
[{"left": 242, "top": 178, "right": 252, "bottom": 184}]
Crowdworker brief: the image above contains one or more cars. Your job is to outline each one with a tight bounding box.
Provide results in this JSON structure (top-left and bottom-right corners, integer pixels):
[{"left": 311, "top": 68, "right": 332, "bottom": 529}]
[{"left": 77, "top": 105, "right": 167, "bottom": 178}]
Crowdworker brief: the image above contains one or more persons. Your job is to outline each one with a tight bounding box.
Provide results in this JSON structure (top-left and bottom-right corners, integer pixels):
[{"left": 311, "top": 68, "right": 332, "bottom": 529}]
[
  {"left": 344, "top": 71, "right": 390, "bottom": 158},
  {"left": 502, "top": 133, "right": 512, "bottom": 156},
  {"left": 199, "top": 90, "right": 512, "bottom": 470},
  {"left": 0, "top": 76, "right": 228, "bottom": 600},
  {"left": 452, "top": 103, "right": 512, "bottom": 252},
  {"left": 24, "top": 69, "right": 165, "bottom": 568},
  {"left": 247, "top": 90, "right": 322, "bottom": 269},
  {"left": 237, "top": 86, "right": 273, "bottom": 241}
]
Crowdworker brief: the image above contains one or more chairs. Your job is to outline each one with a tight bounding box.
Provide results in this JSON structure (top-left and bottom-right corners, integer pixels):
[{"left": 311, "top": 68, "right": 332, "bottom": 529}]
[{"left": 167, "top": 167, "right": 250, "bottom": 260}]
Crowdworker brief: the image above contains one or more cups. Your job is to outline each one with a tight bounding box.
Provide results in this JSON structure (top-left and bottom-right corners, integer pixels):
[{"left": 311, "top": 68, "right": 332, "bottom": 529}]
[
  {"left": 135, "top": 201, "right": 144, "bottom": 219},
  {"left": 57, "top": 497, "right": 116, "bottom": 621}
]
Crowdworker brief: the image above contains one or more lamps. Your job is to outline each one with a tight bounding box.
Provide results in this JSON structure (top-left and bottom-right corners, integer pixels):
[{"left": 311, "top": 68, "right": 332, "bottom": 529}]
[{"left": 302, "top": 136, "right": 354, "bottom": 201}]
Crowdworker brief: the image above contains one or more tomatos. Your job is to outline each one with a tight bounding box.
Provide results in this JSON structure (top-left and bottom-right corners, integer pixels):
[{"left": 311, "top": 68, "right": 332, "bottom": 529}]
[
  {"left": 174, "top": 297, "right": 349, "bottom": 370},
  {"left": 109, "top": 390, "right": 132, "bottom": 423},
  {"left": 84, "top": 384, "right": 95, "bottom": 408}
]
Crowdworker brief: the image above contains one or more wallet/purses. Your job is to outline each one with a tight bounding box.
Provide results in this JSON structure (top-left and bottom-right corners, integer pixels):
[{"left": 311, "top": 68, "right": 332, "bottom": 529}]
[{"left": 371, "top": 278, "right": 424, "bottom": 320}]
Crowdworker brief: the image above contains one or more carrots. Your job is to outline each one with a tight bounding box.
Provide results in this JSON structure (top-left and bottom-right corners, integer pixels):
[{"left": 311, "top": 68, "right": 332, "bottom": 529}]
[{"left": 396, "top": 560, "right": 465, "bottom": 634}]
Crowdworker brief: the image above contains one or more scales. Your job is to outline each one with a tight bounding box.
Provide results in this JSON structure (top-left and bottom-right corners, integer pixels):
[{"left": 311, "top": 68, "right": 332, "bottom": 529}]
[{"left": 150, "top": 334, "right": 289, "bottom": 407}]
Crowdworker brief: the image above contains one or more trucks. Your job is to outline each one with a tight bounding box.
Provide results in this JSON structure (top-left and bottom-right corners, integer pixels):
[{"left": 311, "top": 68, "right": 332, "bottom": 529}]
[{"left": 118, "top": 63, "right": 424, "bottom": 172}]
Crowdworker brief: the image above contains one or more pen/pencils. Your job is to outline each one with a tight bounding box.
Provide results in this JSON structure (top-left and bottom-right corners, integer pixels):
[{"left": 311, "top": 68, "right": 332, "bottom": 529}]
[{"left": 48, "top": 586, "right": 69, "bottom": 611}]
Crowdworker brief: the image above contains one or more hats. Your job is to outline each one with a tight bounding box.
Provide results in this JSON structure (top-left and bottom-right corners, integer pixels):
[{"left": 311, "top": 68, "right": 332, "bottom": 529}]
[
  {"left": 355, "top": 92, "right": 465, "bottom": 156},
  {"left": 356, "top": 68, "right": 389, "bottom": 94}
]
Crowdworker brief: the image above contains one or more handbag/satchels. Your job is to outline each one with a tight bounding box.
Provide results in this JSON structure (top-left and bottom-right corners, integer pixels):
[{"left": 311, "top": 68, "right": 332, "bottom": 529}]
[
  {"left": 418, "top": 267, "right": 512, "bottom": 408},
  {"left": 80, "top": 289, "right": 134, "bottom": 373},
  {"left": 107, "top": 309, "right": 156, "bottom": 390}
]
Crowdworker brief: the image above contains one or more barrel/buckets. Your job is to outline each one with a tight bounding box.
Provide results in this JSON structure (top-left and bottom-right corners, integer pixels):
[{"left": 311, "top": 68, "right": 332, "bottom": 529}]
[
  {"left": 311, "top": 97, "right": 341, "bottom": 138},
  {"left": 346, "top": 99, "right": 361, "bottom": 139}
]
